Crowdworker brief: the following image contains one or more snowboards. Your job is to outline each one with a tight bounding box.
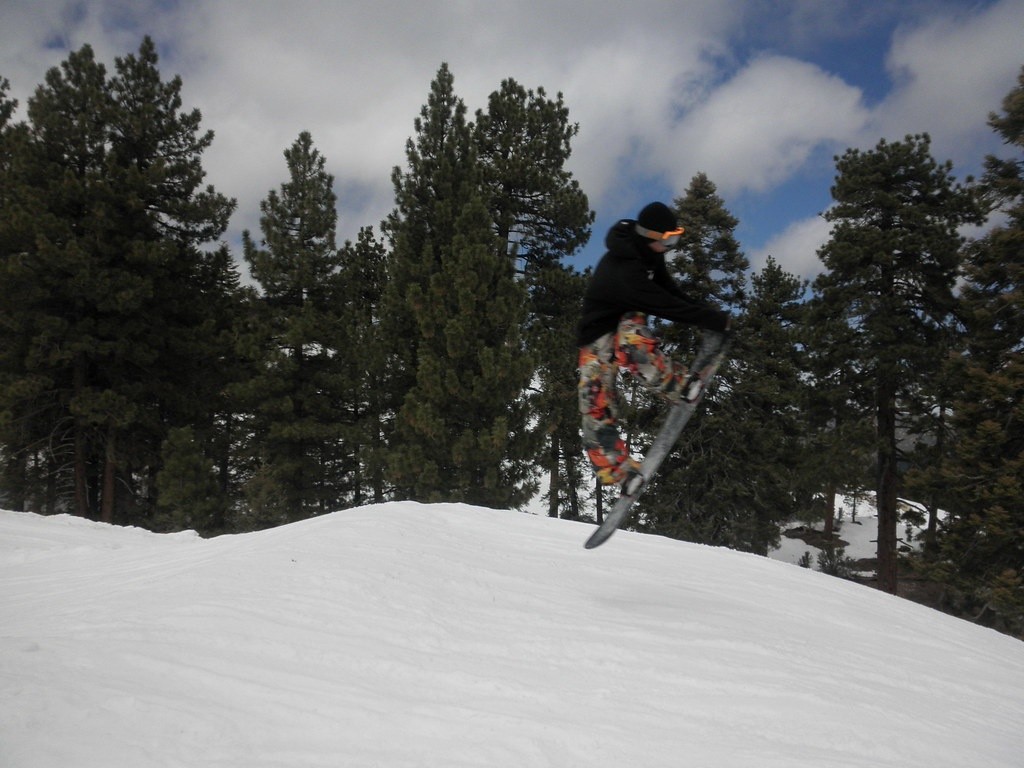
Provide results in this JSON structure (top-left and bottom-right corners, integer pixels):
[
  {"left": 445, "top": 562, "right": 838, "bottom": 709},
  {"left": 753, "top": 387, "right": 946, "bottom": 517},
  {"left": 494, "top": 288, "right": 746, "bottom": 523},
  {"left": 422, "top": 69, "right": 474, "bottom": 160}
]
[{"left": 582, "top": 323, "right": 739, "bottom": 552}]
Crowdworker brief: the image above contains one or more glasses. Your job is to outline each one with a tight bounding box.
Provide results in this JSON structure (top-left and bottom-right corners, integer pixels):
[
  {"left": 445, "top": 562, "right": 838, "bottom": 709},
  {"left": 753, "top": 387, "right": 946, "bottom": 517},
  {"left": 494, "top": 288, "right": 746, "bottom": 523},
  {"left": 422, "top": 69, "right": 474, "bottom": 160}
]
[{"left": 662, "top": 228, "right": 684, "bottom": 247}]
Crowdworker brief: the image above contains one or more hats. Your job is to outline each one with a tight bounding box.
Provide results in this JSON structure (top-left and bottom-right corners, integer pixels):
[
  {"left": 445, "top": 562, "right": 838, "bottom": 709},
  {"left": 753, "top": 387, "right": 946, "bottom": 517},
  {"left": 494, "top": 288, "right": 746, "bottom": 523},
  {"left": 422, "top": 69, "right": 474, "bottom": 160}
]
[{"left": 637, "top": 202, "right": 677, "bottom": 243}]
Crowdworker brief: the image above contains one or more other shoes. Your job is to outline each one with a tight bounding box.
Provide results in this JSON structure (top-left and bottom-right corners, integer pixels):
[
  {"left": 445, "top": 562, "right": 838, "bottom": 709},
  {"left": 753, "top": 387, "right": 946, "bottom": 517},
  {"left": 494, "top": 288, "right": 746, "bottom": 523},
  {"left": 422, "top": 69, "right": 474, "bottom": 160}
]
[
  {"left": 666, "top": 374, "right": 702, "bottom": 405},
  {"left": 619, "top": 466, "right": 644, "bottom": 496}
]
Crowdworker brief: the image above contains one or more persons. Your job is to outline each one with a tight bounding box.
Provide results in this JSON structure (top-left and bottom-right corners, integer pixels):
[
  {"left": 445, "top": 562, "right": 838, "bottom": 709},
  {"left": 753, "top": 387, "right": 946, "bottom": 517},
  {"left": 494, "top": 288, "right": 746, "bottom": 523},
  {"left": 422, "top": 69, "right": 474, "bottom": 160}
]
[{"left": 576, "top": 202, "right": 736, "bottom": 498}]
